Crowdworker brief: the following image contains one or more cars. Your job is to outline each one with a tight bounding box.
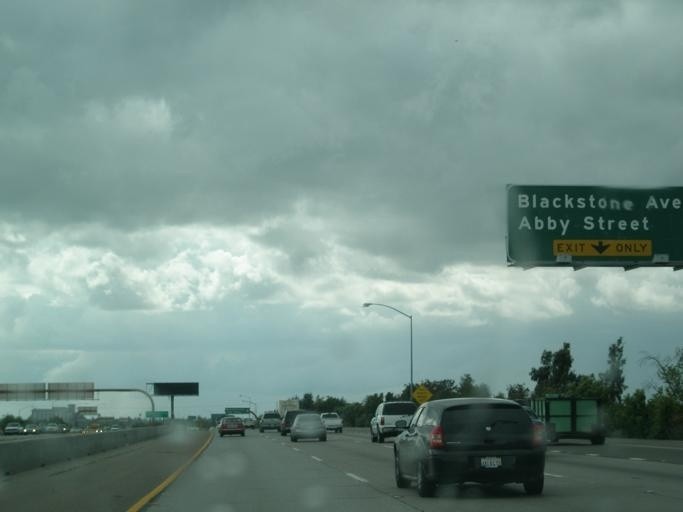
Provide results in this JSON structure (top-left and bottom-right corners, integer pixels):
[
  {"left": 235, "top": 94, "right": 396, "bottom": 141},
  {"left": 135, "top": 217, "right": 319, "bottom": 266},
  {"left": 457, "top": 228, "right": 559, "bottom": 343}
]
[
  {"left": 4, "top": 421, "right": 121, "bottom": 433},
  {"left": 217, "top": 409, "right": 344, "bottom": 443}
]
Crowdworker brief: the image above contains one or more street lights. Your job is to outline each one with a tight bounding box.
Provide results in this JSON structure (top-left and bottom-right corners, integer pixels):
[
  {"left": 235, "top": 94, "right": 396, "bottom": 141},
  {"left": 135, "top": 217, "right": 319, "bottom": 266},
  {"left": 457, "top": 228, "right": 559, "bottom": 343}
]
[
  {"left": 240, "top": 398, "right": 257, "bottom": 418},
  {"left": 238, "top": 393, "right": 250, "bottom": 419},
  {"left": 362, "top": 303, "right": 412, "bottom": 400}
]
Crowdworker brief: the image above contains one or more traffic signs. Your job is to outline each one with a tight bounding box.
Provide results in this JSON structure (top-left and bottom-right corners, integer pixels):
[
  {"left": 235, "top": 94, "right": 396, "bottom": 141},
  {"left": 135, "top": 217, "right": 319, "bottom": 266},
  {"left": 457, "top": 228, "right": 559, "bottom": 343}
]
[{"left": 505, "top": 183, "right": 682, "bottom": 270}]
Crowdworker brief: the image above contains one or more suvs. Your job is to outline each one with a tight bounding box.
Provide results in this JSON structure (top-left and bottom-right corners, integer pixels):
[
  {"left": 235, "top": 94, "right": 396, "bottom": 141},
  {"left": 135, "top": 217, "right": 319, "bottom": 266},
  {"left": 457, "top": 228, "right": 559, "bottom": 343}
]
[{"left": 370, "top": 396, "right": 547, "bottom": 496}]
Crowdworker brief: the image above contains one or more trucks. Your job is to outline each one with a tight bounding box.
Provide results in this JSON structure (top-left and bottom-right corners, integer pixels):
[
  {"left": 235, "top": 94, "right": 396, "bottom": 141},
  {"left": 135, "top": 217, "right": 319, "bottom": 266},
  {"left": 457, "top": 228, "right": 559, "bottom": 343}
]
[{"left": 524, "top": 393, "right": 606, "bottom": 445}]
